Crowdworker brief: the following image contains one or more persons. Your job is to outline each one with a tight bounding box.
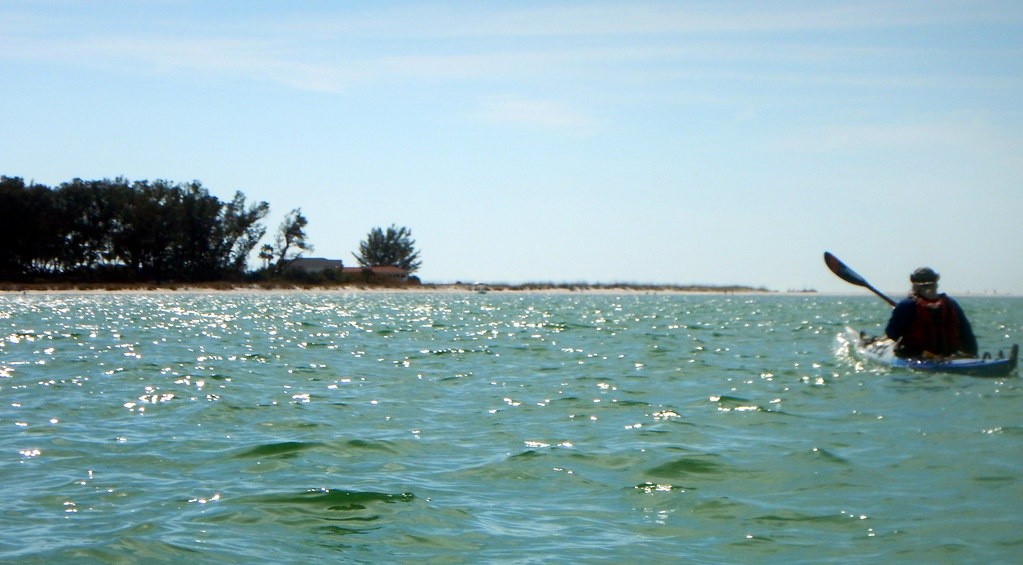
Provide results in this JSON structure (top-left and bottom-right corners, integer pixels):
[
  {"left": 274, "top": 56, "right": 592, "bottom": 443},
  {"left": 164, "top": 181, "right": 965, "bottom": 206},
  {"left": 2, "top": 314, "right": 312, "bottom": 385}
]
[{"left": 860, "top": 267, "right": 978, "bottom": 361}]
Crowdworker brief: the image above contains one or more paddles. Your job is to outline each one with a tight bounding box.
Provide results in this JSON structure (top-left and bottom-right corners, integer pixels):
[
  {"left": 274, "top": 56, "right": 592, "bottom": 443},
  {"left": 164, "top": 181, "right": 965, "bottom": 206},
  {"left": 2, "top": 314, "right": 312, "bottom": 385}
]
[{"left": 823, "top": 250, "right": 897, "bottom": 307}]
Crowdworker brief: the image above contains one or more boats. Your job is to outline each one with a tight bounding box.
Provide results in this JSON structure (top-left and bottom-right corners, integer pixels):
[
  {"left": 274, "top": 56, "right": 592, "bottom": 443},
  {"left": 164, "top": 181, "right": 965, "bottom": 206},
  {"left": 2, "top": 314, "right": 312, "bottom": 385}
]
[{"left": 844, "top": 325, "right": 1019, "bottom": 377}]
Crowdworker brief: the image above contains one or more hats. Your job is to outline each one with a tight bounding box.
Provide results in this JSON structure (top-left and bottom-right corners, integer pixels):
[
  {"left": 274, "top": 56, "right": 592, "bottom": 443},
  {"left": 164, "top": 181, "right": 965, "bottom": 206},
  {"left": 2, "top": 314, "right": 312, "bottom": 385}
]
[{"left": 910, "top": 267, "right": 940, "bottom": 286}]
